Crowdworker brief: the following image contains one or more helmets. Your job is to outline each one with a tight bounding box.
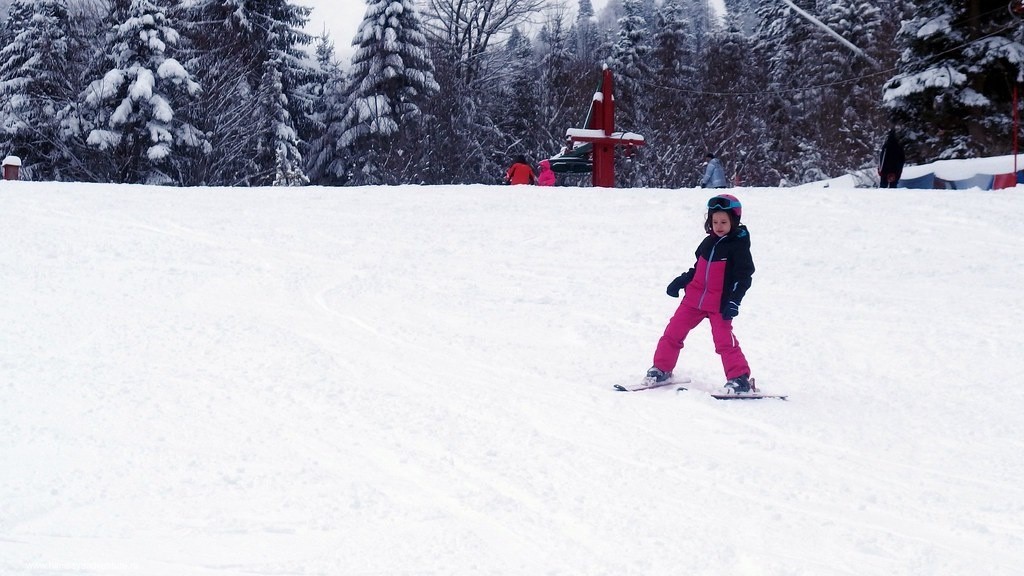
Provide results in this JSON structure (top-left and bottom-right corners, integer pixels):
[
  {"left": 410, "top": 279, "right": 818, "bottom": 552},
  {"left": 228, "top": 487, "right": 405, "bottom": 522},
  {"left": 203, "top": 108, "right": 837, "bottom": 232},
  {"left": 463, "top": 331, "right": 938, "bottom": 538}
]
[{"left": 706, "top": 194, "right": 742, "bottom": 232}]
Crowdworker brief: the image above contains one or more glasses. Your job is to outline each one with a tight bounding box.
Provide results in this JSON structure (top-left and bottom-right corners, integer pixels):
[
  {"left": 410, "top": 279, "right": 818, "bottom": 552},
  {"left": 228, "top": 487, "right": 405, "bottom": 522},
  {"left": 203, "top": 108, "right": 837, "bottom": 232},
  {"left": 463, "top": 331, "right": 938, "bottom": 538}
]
[{"left": 707, "top": 196, "right": 733, "bottom": 210}]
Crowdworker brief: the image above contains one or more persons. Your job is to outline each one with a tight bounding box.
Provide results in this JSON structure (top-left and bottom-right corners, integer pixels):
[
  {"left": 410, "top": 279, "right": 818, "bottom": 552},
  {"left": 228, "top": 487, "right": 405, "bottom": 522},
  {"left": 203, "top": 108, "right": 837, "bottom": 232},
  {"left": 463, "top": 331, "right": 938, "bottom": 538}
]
[
  {"left": 506, "top": 155, "right": 535, "bottom": 185},
  {"left": 538, "top": 160, "right": 555, "bottom": 186},
  {"left": 647, "top": 194, "right": 755, "bottom": 392},
  {"left": 879, "top": 130, "right": 904, "bottom": 188},
  {"left": 702, "top": 153, "right": 726, "bottom": 188}
]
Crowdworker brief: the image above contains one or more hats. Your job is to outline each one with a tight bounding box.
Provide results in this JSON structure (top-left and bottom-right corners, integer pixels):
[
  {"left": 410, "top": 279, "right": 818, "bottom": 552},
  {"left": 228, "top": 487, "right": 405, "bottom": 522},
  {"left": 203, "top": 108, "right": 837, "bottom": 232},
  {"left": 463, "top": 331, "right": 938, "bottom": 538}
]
[
  {"left": 705, "top": 153, "right": 716, "bottom": 159},
  {"left": 515, "top": 154, "right": 526, "bottom": 162}
]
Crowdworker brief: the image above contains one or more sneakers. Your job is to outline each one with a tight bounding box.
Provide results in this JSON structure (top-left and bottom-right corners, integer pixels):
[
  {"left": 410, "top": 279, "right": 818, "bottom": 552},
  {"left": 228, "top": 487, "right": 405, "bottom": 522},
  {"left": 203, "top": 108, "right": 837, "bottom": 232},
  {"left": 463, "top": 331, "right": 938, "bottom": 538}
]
[
  {"left": 643, "top": 366, "right": 674, "bottom": 384},
  {"left": 720, "top": 376, "right": 750, "bottom": 394}
]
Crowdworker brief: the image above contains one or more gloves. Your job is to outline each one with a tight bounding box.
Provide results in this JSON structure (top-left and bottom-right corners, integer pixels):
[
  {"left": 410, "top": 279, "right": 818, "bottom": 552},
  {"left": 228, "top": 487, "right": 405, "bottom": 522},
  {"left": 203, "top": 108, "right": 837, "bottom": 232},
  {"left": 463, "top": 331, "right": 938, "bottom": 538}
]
[
  {"left": 722, "top": 298, "right": 741, "bottom": 321},
  {"left": 701, "top": 183, "right": 707, "bottom": 188},
  {"left": 666, "top": 272, "right": 689, "bottom": 297}
]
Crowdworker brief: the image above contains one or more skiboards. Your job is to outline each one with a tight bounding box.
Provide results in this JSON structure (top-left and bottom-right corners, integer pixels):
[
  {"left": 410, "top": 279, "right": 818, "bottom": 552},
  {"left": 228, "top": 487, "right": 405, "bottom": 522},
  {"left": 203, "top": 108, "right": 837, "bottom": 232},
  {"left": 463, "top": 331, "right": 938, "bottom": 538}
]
[{"left": 612, "top": 377, "right": 790, "bottom": 400}]
